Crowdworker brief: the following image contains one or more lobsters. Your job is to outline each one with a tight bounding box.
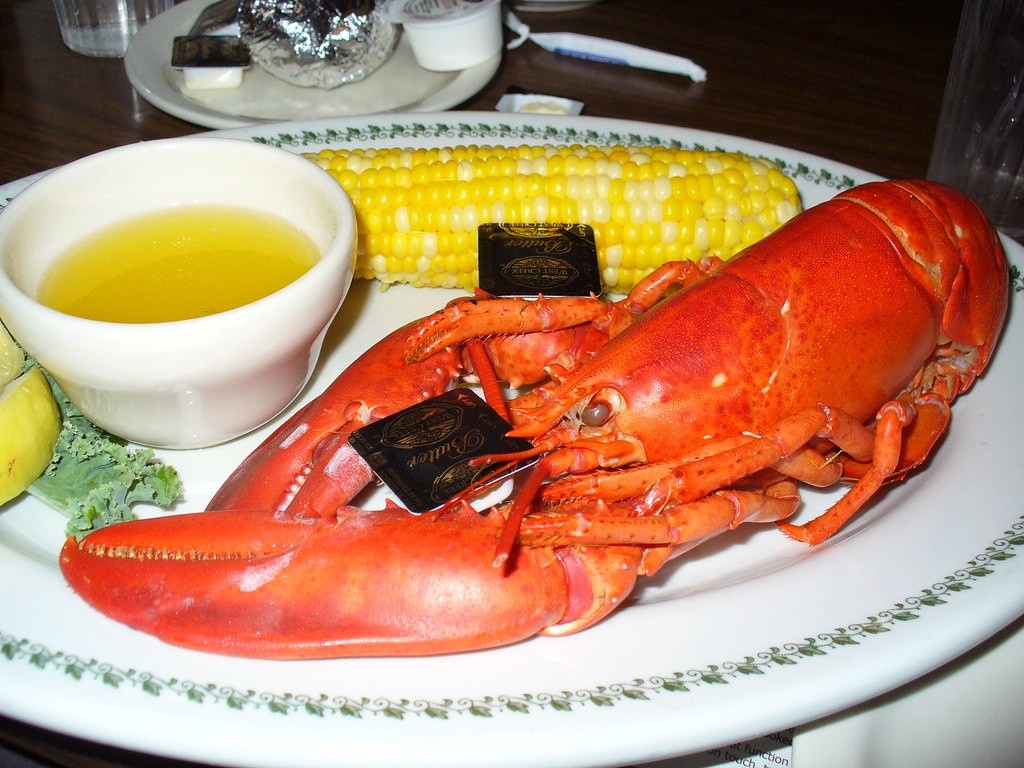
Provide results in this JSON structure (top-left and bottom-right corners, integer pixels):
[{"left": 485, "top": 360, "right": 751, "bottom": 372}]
[{"left": 56, "top": 176, "right": 1010, "bottom": 660}]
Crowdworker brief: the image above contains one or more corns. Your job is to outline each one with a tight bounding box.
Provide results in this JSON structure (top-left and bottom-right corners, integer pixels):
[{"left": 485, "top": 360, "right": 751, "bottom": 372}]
[{"left": 290, "top": 143, "right": 803, "bottom": 295}]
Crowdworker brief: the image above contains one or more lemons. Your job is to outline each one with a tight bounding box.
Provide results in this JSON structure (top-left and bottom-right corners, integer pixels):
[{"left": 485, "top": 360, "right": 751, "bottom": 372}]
[{"left": 1, "top": 320, "right": 63, "bottom": 509}]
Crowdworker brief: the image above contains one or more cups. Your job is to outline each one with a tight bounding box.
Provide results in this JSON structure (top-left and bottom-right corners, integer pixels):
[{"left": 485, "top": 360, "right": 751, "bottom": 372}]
[
  {"left": 925, "top": 1, "right": 1023, "bottom": 240},
  {"left": 52, "top": 0, "right": 180, "bottom": 59}
]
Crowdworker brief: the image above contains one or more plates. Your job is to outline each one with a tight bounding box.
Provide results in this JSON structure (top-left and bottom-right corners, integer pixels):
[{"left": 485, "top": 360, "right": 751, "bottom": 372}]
[
  {"left": 0, "top": 112, "right": 1024, "bottom": 767},
  {"left": 124, "top": 0, "right": 502, "bottom": 134}
]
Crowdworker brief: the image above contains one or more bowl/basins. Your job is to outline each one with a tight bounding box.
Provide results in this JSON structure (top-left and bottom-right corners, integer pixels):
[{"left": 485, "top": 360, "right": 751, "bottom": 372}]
[{"left": 0, "top": 139, "right": 358, "bottom": 449}]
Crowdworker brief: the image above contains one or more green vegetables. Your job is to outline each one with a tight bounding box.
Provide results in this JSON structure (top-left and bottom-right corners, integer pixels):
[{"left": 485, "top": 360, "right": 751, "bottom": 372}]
[{"left": 13, "top": 337, "right": 185, "bottom": 541}]
